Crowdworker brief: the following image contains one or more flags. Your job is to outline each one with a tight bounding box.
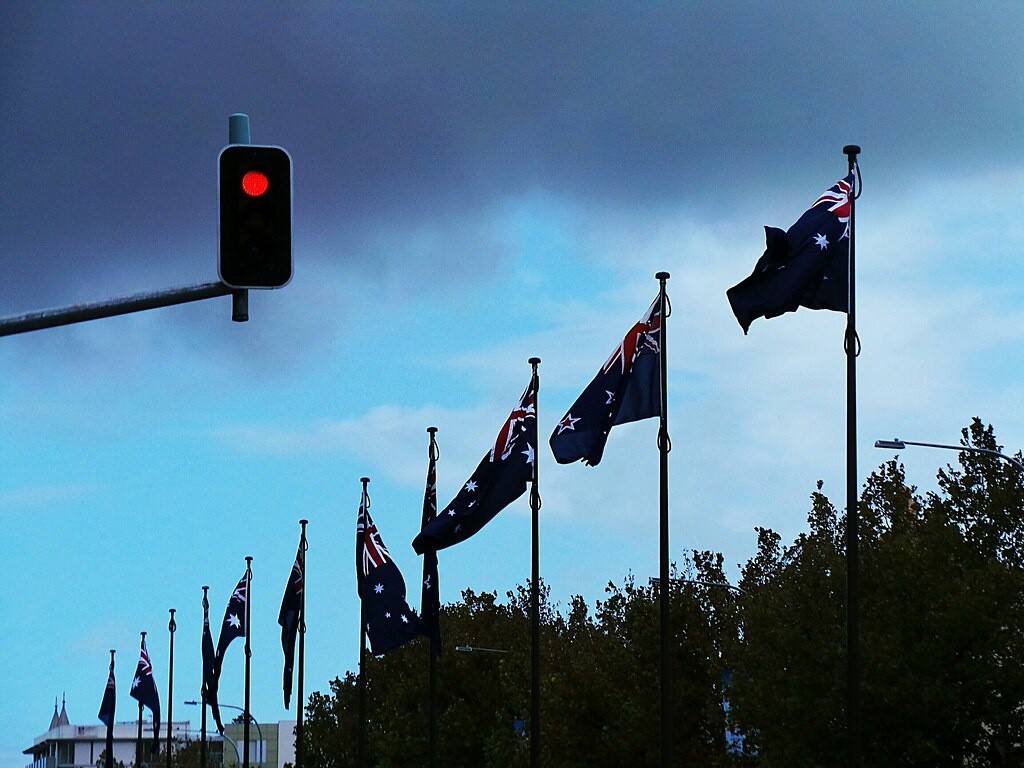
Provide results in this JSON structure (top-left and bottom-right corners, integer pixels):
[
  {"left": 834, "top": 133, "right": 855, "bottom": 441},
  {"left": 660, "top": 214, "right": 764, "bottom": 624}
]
[
  {"left": 130, "top": 640, "right": 160, "bottom": 737},
  {"left": 727, "top": 173, "right": 854, "bottom": 336},
  {"left": 548, "top": 292, "right": 662, "bottom": 466},
  {"left": 356, "top": 493, "right": 421, "bottom": 658},
  {"left": 421, "top": 437, "right": 441, "bottom": 639},
  {"left": 202, "top": 569, "right": 248, "bottom": 736},
  {"left": 98, "top": 660, "right": 116, "bottom": 740},
  {"left": 412, "top": 373, "right": 539, "bottom": 556},
  {"left": 278, "top": 534, "right": 305, "bottom": 711}
]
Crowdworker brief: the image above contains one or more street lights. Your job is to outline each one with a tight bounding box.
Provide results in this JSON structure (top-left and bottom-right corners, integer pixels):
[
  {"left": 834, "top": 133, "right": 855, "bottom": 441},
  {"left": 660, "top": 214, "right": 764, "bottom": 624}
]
[
  {"left": 649, "top": 577, "right": 750, "bottom": 595},
  {"left": 874, "top": 438, "right": 1024, "bottom": 472},
  {"left": 184, "top": 700, "right": 262, "bottom": 768},
  {"left": 143, "top": 727, "right": 240, "bottom": 768},
  {"left": 456, "top": 646, "right": 509, "bottom": 653}
]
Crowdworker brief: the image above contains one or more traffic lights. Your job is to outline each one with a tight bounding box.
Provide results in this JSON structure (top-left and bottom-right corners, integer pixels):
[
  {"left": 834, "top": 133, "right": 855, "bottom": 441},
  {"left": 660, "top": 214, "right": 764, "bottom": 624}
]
[{"left": 217, "top": 145, "right": 294, "bottom": 290}]
